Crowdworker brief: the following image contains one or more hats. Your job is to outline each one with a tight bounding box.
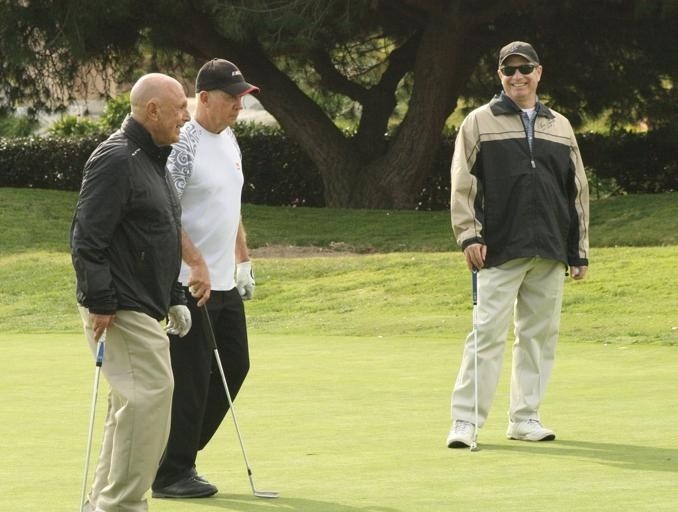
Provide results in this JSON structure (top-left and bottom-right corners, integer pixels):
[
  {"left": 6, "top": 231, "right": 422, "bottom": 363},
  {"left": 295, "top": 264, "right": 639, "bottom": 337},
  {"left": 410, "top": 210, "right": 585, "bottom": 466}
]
[
  {"left": 194, "top": 57, "right": 261, "bottom": 98},
  {"left": 498, "top": 41, "right": 540, "bottom": 67}
]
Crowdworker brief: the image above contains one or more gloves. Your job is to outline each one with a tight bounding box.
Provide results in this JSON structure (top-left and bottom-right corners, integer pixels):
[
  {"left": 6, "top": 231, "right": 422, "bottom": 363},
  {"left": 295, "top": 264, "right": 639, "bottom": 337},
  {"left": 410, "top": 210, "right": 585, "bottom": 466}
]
[
  {"left": 164, "top": 299, "right": 192, "bottom": 338},
  {"left": 235, "top": 260, "right": 256, "bottom": 302}
]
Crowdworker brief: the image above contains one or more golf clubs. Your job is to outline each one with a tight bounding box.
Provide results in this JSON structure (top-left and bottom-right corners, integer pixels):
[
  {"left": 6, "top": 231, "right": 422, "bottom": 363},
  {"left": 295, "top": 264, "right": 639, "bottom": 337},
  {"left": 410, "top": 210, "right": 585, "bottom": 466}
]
[
  {"left": 470, "top": 270, "right": 478, "bottom": 451},
  {"left": 200, "top": 302, "right": 284, "bottom": 499}
]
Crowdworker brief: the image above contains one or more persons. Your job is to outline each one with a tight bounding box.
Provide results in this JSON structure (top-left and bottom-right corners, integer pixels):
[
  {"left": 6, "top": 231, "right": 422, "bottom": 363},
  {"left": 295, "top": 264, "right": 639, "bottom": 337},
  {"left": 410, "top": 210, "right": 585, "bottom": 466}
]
[
  {"left": 442, "top": 39, "right": 593, "bottom": 449},
  {"left": 67, "top": 70, "right": 197, "bottom": 512},
  {"left": 147, "top": 58, "right": 261, "bottom": 500}
]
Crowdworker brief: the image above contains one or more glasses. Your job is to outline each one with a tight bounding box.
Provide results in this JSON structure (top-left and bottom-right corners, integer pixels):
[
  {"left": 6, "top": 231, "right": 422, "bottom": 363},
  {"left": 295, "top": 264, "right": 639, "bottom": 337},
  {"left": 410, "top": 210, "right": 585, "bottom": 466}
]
[{"left": 501, "top": 64, "right": 536, "bottom": 76}]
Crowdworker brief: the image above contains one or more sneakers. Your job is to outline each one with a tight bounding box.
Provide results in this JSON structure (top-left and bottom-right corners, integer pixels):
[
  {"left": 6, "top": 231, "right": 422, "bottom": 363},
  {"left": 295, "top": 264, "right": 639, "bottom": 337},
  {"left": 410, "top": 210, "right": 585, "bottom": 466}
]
[
  {"left": 506, "top": 416, "right": 555, "bottom": 442},
  {"left": 152, "top": 466, "right": 219, "bottom": 499},
  {"left": 447, "top": 419, "right": 479, "bottom": 449}
]
